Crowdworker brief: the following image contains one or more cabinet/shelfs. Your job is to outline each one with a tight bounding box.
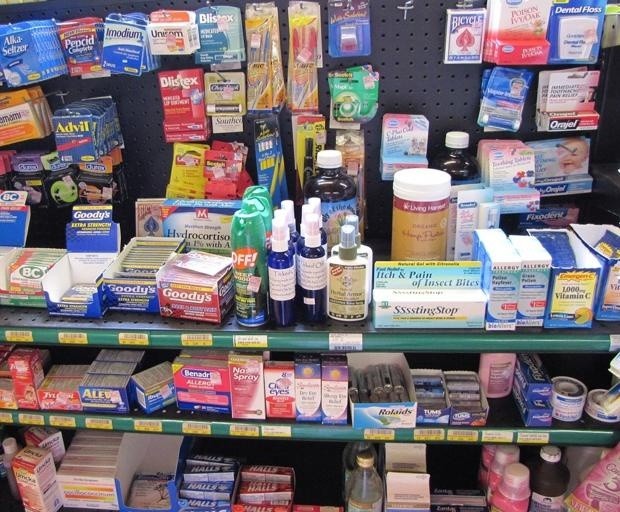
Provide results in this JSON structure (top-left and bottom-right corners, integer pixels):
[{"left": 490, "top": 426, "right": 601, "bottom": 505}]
[{"left": 0, "top": 306, "right": 620, "bottom": 512}]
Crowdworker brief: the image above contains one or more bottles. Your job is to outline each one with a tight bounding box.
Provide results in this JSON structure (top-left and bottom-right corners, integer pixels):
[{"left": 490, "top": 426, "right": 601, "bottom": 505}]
[
  {"left": 342, "top": 441, "right": 384, "bottom": 512},
  {"left": 231, "top": 149, "right": 374, "bottom": 326},
  {"left": 480, "top": 443, "right": 603, "bottom": 511},
  {"left": 2, "top": 438, "right": 23, "bottom": 500},
  {"left": 430, "top": 130, "right": 482, "bottom": 185},
  {"left": 391, "top": 168, "right": 452, "bottom": 263}
]
